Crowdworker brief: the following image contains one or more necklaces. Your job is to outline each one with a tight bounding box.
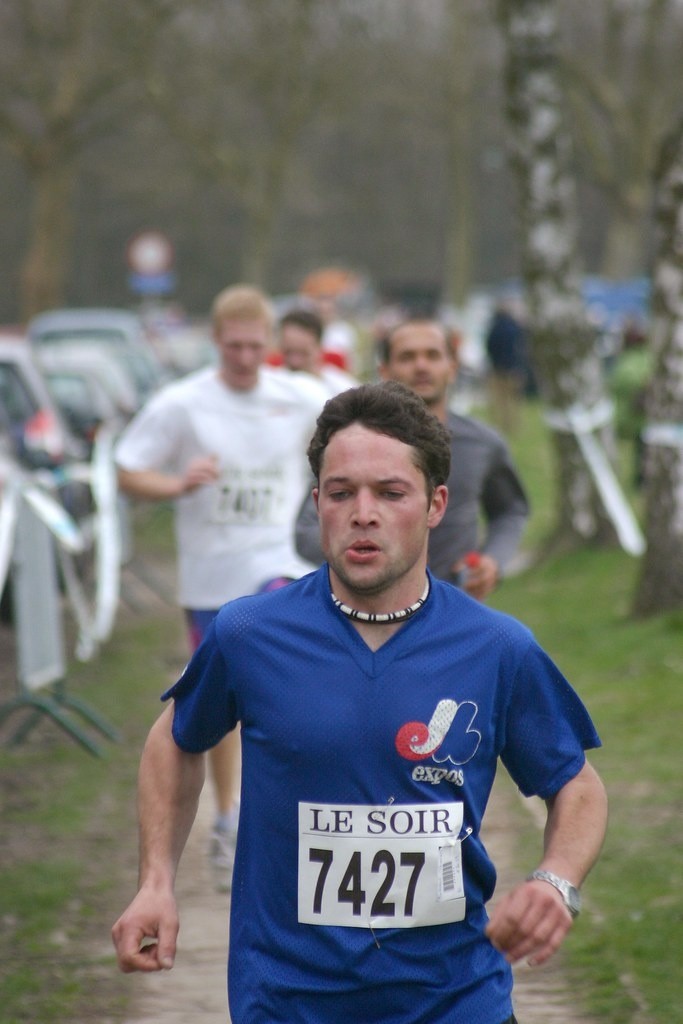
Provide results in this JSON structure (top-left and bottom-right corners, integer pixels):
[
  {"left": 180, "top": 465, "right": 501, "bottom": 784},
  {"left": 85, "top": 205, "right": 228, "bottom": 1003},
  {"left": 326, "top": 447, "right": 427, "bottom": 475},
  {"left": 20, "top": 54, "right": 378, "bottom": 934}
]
[{"left": 330, "top": 577, "right": 429, "bottom": 624}]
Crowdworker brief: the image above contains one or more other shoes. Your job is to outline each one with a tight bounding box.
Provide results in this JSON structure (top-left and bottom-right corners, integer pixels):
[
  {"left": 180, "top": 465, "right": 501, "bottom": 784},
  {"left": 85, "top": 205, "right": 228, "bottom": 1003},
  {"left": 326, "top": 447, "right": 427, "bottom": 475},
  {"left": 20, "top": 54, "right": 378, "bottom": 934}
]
[{"left": 212, "top": 800, "right": 240, "bottom": 893}]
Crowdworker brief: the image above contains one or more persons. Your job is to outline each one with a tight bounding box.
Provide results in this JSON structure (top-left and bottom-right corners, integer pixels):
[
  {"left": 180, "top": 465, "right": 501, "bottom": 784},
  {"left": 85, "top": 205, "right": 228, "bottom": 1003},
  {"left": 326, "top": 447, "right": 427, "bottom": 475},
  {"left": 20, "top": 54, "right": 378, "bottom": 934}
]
[
  {"left": 312, "top": 297, "right": 352, "bottom": 354},
  {"left": 293, "top": 318, "right": 532, "bottom": 601},
  {"left": 485, "top": 309, "right": 524, "bottom": 430},
  {"left": 111, "top": 380, "right": 609, "bottom": 1024},
  {"left": 275, "top": 311, "right": 361, "bottom": 394},
  {"left": 112, "top": 285, "right": 335, "bottom": 895}
]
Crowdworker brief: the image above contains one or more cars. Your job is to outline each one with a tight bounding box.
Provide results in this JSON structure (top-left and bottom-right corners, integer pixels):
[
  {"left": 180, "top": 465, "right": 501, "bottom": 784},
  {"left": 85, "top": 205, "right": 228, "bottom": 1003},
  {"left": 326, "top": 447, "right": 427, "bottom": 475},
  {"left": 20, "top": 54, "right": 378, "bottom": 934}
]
[{"left": 0, "top": 308, "right": 211, "bottom": 625}]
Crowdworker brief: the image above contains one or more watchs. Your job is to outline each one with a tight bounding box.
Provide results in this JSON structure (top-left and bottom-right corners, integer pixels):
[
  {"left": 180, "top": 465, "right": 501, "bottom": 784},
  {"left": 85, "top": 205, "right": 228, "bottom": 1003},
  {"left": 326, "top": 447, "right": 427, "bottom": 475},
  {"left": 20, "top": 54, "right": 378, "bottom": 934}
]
[{"left": 526, "top": 870, "right": 582, "bottom": 919}]
